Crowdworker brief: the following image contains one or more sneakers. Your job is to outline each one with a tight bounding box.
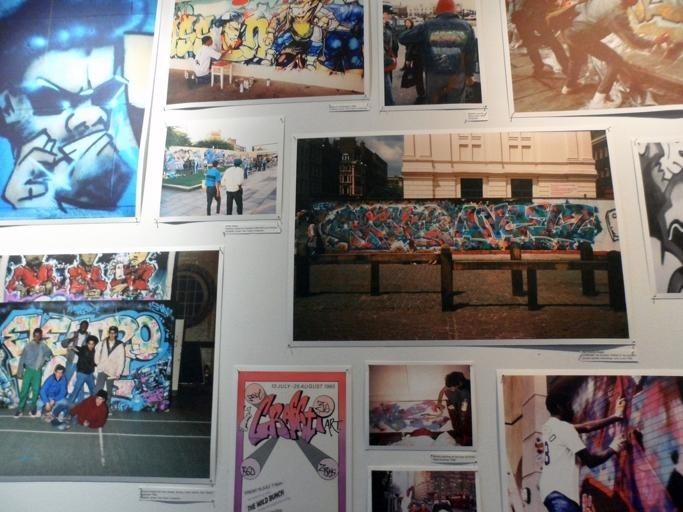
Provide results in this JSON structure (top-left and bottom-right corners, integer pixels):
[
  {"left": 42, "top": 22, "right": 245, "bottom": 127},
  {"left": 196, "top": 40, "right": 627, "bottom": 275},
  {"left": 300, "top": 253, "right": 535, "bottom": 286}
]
[
  {"left": 561, "top": 79, "right": 585, "bottom": 95},
  {"left": 594, "top": 98, "right": 613, "bottom": 106},
  {"left": 188, "top": 78, "right": 193, "bottom": 88},
  {"left": 15, "top": 410, "right": 41, "bottom": 417},
  {"left": 44, "top": 411, "right": 66, "bottom": 430}
]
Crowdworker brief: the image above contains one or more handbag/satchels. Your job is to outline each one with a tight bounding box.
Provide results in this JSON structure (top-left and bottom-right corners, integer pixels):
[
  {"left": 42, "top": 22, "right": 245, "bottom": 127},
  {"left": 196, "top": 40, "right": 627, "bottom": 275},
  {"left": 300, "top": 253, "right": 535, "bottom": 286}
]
[
  {"left": 401, "top": 60, "right": 418, "bottom": 88},
  {"left": 385, "top": 48, "right": 397, "bottom": 72}
]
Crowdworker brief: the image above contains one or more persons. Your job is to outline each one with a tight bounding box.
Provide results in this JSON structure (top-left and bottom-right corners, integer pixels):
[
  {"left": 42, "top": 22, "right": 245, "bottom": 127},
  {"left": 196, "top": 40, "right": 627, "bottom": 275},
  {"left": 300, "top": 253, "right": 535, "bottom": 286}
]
[
  {"left": 561, "top": 0, "right": 671, "bottom": 107},
  {"left": 188, "top": 35, "right": 231, "bottom": 88},
  {"left": 220, "top": 159, "right": 245, "bottom": 216},
  {"left": 396, "top": 0, "right": 476, "bottom": 105},
  {"left": 204, "top": 159, "right": 222, "bottom": 216},
  {"left": 538, "top": 384, "right": 627, "bottom": 510},
  {"left": 383, "top": 4, "right": 398, "bottom": 103},
  {"left": 436, "top": 369, "right": 469, "bottom": 413},
  {"left": 15, "top": 319, "right": 127, "bottom": 429},
  {"left": 400, "top": 19, "right": 415, "bottom": 70}
]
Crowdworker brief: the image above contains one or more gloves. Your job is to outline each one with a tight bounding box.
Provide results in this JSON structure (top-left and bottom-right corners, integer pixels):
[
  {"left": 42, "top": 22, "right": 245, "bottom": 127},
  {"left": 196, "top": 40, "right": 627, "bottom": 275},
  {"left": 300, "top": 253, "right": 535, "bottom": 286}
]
[
  {"left": 615, "top": 396, "right": 625, "bottom": 418},
  {"left": 608, "top": 433, "right": 627, "bottom": 453}
]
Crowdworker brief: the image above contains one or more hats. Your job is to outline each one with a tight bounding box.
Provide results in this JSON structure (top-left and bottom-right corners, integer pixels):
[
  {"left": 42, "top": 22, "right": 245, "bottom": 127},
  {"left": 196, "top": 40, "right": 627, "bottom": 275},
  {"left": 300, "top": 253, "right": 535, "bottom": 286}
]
[
  {"left": 384, "top": 5, "right": 397, "bottom": 15},
  {"left": 437, "top": 0, "right": 454, "bottom": 13},
  {"left": 234, "top": 159, "right": 242, "bottom": 165},
  {"left": 95, "top": 389, "right": 108, "bottom": 399}
]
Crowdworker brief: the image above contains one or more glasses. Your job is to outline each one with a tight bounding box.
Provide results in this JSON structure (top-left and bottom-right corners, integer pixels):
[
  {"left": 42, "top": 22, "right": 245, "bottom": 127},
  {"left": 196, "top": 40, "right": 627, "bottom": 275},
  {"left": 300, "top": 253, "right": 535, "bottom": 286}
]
[{"left": 16, "top": 76, "right": 129, "bottom": 116}]
